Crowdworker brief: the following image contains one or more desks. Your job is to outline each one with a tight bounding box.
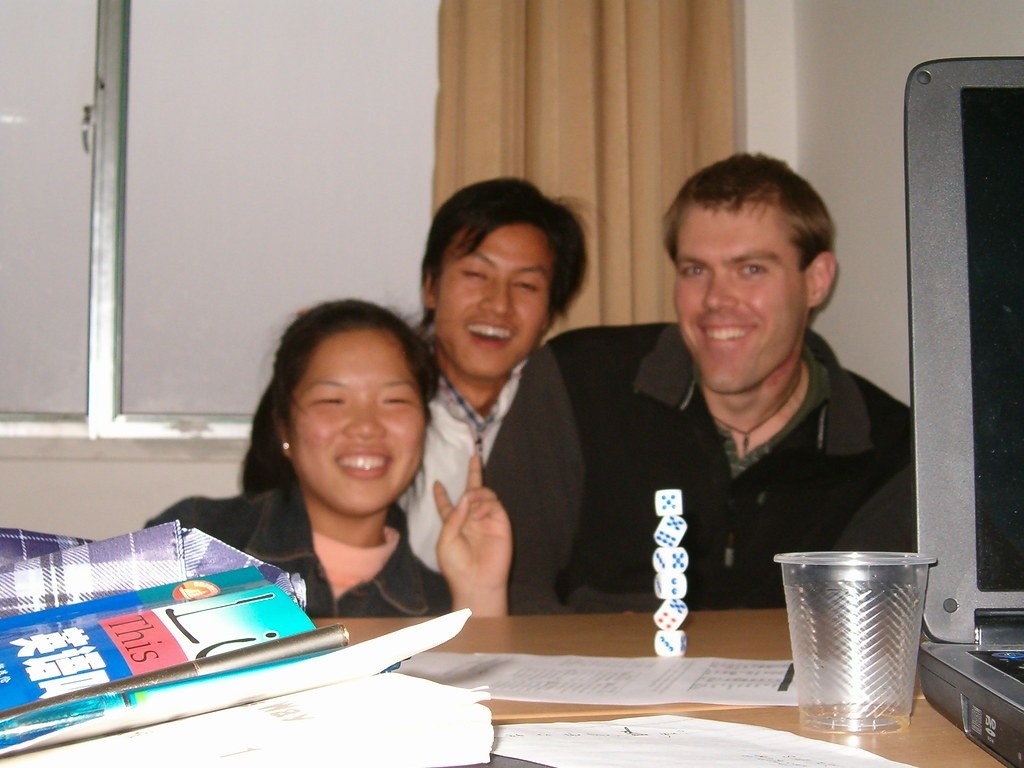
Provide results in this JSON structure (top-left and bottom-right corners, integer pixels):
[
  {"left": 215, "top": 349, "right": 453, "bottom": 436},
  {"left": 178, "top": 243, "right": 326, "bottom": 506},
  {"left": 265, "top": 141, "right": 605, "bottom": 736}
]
[{"left": 310, "top": 609, "right": 1003, "bottom": 768}]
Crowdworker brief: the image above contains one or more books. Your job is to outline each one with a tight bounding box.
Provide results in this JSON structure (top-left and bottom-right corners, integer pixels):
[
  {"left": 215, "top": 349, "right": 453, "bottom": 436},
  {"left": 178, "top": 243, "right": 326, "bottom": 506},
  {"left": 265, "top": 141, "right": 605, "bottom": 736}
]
[{"left": 0, "top": 566, "right": 497, "bottom": 768}]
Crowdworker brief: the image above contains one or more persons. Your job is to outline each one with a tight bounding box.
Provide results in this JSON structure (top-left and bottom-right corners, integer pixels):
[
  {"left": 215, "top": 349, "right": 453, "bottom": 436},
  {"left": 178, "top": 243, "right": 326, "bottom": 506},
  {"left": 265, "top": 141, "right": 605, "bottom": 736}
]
[
  {"left": 397, "top": 178, "right": 595, "bottom": 576},
  {"left": 483, "top": 150, "right": 916, "bottom": 617},
  {"left": 142, "top": 299, "right": 512, "bottom": 618}
]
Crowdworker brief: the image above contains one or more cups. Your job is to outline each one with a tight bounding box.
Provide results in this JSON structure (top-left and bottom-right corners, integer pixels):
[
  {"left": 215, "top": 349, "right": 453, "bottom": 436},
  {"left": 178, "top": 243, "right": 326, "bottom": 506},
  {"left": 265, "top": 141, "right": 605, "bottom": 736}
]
[{"left": 774, "top": 551, "right": 938, "bottom": 734}]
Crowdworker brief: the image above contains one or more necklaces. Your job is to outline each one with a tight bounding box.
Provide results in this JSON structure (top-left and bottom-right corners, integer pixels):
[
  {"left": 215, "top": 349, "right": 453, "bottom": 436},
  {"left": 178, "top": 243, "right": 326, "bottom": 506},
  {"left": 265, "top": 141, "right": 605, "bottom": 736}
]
[{"left": 709, "top": 362, "right": 803, "bottom": 453}]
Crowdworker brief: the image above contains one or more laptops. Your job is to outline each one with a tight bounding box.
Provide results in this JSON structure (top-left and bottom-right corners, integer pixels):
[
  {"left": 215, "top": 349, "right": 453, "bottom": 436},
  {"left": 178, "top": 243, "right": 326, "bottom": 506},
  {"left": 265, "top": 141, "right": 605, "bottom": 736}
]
[{"left": 904, "top": 56, "right": 1024, "bottom": 768}]
